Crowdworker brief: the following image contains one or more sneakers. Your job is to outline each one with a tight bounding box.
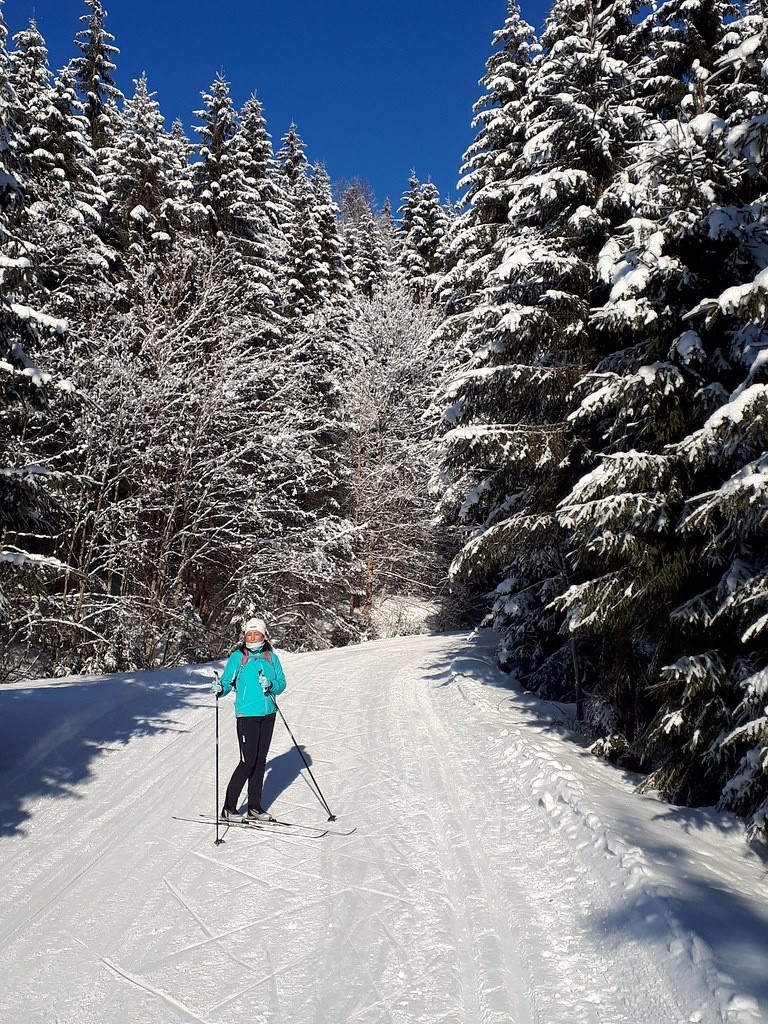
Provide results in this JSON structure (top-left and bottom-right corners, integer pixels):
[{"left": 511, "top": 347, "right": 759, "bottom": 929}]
[
  {"left": 248, "top": 804, "right": 272, "bottom": 821},
  {"left": 220, "top": 807, "right": 245, "bottom": 823}
]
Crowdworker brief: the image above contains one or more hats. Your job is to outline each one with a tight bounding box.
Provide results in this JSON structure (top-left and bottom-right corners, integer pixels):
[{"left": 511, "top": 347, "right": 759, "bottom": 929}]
[{"left": 245, "top": 618, "right": 266, "bottom": 639}]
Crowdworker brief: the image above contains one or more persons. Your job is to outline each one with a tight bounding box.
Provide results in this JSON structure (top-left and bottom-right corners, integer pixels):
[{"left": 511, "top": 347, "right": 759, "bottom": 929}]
[{"left": 212, "top": 617, "right": 289, "bottom": 824}]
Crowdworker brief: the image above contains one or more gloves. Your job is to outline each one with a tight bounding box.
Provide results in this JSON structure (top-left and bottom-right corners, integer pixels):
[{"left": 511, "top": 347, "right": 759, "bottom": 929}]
[
  {"left": 259, "top": 676, "right": 271, "bottom": 687},
  {"left": 212, "top": 680, "right": 222, "bottom": 693}
]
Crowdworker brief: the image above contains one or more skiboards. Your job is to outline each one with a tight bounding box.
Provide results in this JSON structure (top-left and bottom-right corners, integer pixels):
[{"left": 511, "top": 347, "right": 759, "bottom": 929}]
[
  {"left": 199, "top": 811, "right": 358, "bottom": 836},
  {"left": 172, "top": 814, "right": 330, "bottom": 839}
]
